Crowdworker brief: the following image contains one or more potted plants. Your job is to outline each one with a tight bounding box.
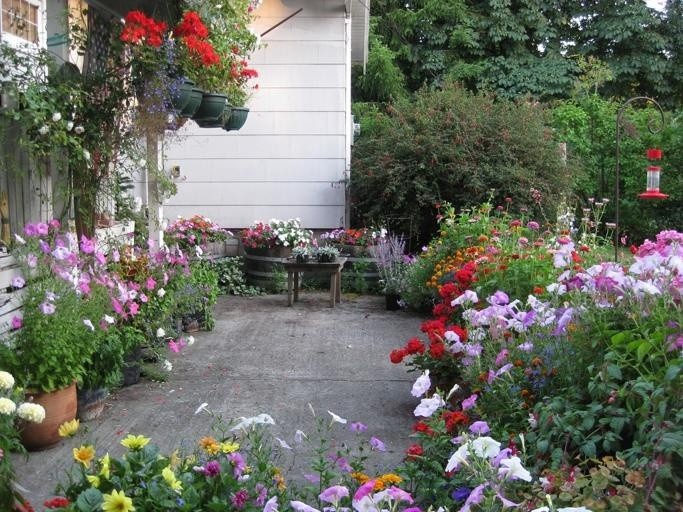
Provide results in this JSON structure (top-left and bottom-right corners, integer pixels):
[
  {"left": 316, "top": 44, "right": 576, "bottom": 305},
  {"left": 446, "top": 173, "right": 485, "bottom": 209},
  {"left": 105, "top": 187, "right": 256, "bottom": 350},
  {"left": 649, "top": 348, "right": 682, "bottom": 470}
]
[{"left": 290, "top": 243, "right": 339, "bottom": 263}]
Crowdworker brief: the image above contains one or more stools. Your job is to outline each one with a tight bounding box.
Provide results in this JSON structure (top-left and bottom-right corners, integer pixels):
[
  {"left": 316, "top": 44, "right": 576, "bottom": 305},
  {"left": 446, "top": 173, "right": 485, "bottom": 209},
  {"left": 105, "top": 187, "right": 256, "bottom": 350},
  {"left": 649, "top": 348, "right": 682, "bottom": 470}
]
[{"left": 281, "top": 256, "right": 347, "bottom": 307}]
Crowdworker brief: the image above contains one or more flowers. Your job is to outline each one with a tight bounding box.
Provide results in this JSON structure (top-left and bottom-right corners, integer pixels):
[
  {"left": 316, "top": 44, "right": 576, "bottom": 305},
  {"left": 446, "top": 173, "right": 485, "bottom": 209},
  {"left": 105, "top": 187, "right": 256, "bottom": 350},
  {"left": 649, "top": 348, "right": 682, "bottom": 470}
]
[
  {"left": 118, "top": 10, "right": 259, "bottom": 113},
  {"left": 239, "top": 218, "right": 312, "bottom": 247},
  {"left": 0, "top": 217, "right": 142, "bottom": 394},
  {"left": 162, "top": 213, "right": 235, "bottom": 247},
  {"left": 321, "top": 226, "right": 406, "bottom": 295}
]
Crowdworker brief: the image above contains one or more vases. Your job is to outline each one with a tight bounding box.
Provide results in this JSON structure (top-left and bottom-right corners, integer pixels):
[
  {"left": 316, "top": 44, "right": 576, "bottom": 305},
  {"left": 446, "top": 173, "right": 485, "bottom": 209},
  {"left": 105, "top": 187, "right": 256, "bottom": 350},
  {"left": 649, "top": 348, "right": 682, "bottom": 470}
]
[
  {"left": 244, "top": 246, "right": 292, "bottom": 258},
  {"left": 195, "top": 239, "right": 238, "bottom": 259},
  {"left": 384, "top": 293, "right": 403, "bottom": 310},
  {"left": 8, "top": 343, "right": 142, "bottom": 451},
  {"left": 133, "top": 80, "right": 248, "bottom": 131}
]
